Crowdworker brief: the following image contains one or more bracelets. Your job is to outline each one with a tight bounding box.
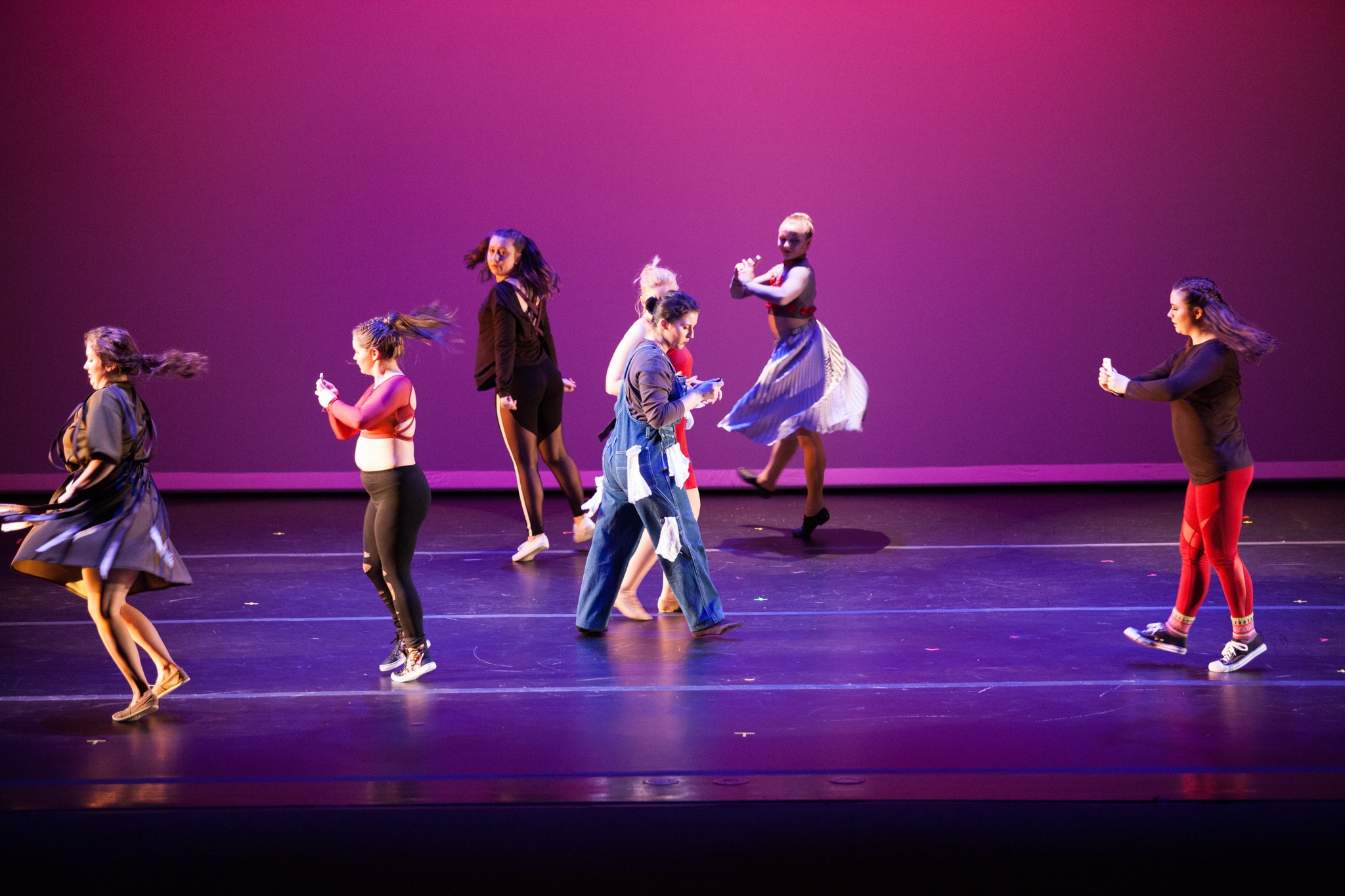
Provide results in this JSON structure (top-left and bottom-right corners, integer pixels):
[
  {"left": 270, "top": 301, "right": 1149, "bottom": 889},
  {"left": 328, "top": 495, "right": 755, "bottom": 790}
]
[{"left": 327, "top": 397, "right": 339, "bottom": 412}]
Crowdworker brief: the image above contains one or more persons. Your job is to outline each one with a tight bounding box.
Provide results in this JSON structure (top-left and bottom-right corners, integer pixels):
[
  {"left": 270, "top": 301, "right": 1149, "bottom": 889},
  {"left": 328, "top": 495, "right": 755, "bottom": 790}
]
[
  {"left": 718, "top": 212, "right": 870, "bottom": 538},
  {"left": 314, "top": 297, "right": 461, "bottom": 683},
  {"left": 576, "top": 253, "right": 743, "bottom": 636},
  {"left": 1097, "top": 276, "right": 1282, "bottom": 673},
  {"left": 1, "top": 326, "right": 209, "bottom": 722},
  {"left": 461, "top": 229, "right": 594, "bottom": 563}
]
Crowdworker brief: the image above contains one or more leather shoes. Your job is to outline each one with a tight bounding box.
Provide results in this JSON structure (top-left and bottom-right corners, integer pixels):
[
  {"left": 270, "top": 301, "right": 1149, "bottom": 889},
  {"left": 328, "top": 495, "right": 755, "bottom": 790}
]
[
  {"left": 792, "top": 507, "right": 830, "bottom": 536},
  {"left": 737, "top": 466, "right": 777, "bottom": 499}
]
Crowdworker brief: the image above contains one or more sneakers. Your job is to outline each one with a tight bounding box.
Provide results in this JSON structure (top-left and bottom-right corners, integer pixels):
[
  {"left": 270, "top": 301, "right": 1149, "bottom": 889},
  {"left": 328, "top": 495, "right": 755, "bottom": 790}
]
[
  {"left": 1208, "top": 633, "right": 1267, "bottom": 672},
  {"left": 391, "top": 643, "right": 437, "bottom": 682},
  {"left": 378, "top": 630, "right": 431, "bottom": 671},
  {"left": 1123, "top": 622, "right": 1188, "bottom": 654}
]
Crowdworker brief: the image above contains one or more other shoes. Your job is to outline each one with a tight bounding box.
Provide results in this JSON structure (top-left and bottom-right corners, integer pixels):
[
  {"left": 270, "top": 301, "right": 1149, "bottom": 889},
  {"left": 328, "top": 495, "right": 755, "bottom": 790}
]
[
  {"left": 512, "top": 535, "right": 549, "bottom": 562},
  {"left": 573, "top": 518, "right": 596, "bottom": 542},
  {"left": 657, "top": 597, "right": 680, "bottom": 612},
  {"left": 112, "top": 694, "right": 158, "bottom": 721},
  {"left": 151, "top": 667, "right": 190, "bottom": 698},
  {"left": 613, "top": 594, "right": 653, "bottom": 620}
]
